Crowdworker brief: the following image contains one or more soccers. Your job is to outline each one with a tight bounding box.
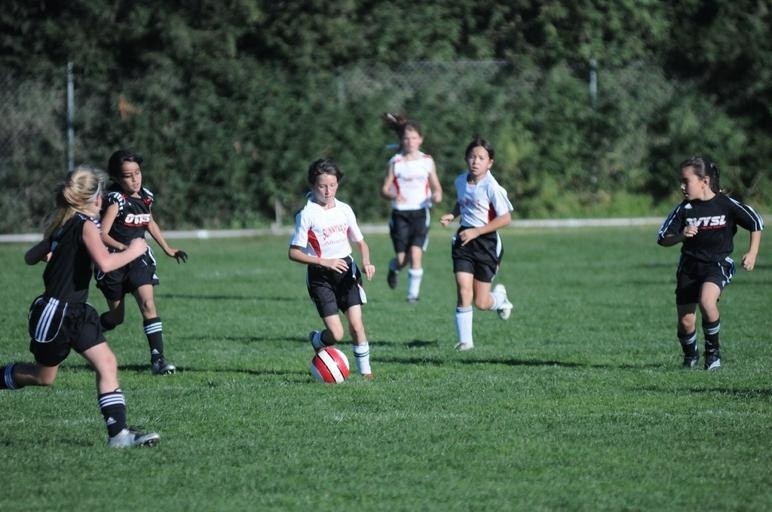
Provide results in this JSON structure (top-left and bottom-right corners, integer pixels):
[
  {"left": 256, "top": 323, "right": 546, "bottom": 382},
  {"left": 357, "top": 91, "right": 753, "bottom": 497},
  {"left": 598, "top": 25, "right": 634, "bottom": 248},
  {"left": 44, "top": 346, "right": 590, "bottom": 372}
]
[{"left": 308, "top": 347, "right": 352, "bottom": 382}]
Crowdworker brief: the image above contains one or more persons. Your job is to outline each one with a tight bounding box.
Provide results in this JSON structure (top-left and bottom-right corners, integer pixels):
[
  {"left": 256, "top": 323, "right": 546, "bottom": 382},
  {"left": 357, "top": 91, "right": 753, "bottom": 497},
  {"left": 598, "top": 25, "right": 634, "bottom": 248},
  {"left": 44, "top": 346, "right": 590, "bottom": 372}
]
[
  {"left": 657, "top": 154, "right": 765, "bottom": 369},
  {"left": 97, "top": 150, "right": 190, "bottom": 375},
  {"left": 288, "top": 159, "right": 379, "bottom": 382},
  {"left": 1, "top": 165, "right": 160, "bottom": 450},
  {"left": 441, "top": 138, "right": 513, "bottom": 353},
  {"left": 380, "top": 113, "right": 441, "bottom": 303}
]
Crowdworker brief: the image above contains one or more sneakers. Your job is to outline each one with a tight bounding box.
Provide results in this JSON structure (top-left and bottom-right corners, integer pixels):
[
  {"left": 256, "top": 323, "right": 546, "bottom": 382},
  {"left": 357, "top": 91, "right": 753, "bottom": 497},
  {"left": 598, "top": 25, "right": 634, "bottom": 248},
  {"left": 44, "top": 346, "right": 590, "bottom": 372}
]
[
  {"left": 703, "top": 348, "right": 722, "bottom": 371},
  {"left": 149, "top": 358, "right": 176, "bottom": 376},
  {"left": 308, "top": 330, "right": 325, "bottom": 352},
  {"left": 493, "top": 284, "right": 513, "bottom": 321},
  {"left": 453, "top": 342, "right": 474, "bottom": 353},
  {"left": 682, "top": 350, "right": 700, "bottom": 369},
  {"left": 387, "top": 258, "right": 400, "bottom": 289},
  {"left": 406, "top": 295, "right": 419, "bottom": 304},
  {"left": 107, "top": 426, "right": 162, "bottom": 449},
  {"left": 361, "top": 373, "right": 374, "bottom": 382}
]
